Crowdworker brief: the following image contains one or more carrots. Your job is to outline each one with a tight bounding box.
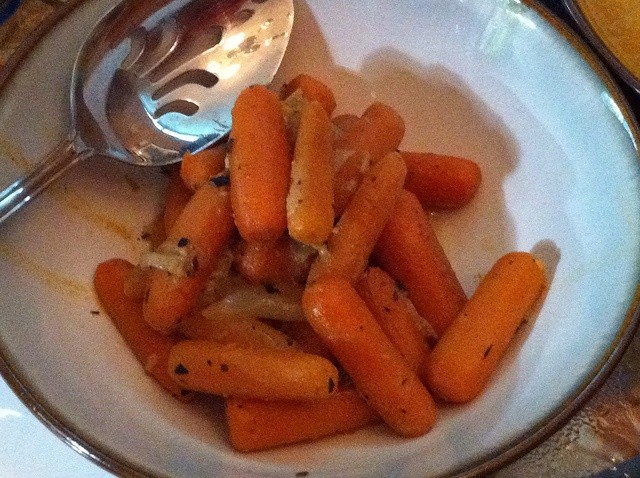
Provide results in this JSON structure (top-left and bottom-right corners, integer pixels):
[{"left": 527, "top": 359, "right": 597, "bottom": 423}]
[{"left": 95, "top": 75, "right": 546, "bottom": 452}]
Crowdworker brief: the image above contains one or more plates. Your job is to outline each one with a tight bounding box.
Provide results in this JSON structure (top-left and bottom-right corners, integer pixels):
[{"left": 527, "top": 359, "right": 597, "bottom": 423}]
[{"left": 0, "top": 1, "right": 640, "bottom": 478}]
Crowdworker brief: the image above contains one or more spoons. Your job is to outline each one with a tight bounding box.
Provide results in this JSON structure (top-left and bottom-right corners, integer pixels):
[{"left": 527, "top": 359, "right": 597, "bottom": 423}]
[{"left": 0, "top": 1, "right": 295, "bottom": 226}]
